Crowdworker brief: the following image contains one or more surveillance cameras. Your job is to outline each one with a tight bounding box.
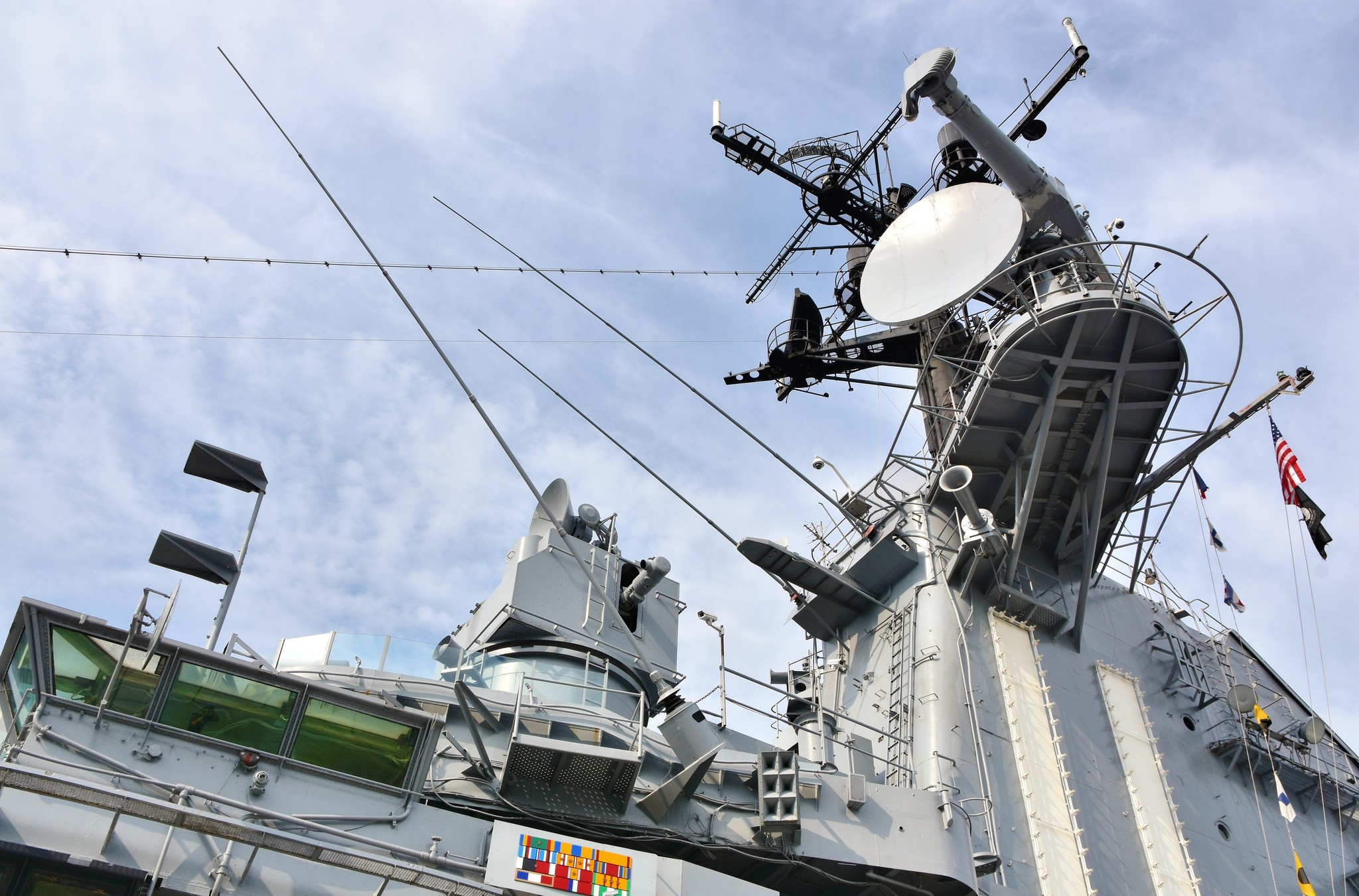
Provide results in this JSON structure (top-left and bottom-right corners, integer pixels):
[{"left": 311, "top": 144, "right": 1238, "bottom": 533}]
[{"left": 812, "top": 458, "right": 824, "bottom": 471}]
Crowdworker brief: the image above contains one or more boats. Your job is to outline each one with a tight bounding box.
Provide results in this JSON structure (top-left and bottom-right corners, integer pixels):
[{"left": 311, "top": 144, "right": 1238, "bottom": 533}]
[{"left": 0, "top": 13, "right": 1359, "bottom": 896}]
